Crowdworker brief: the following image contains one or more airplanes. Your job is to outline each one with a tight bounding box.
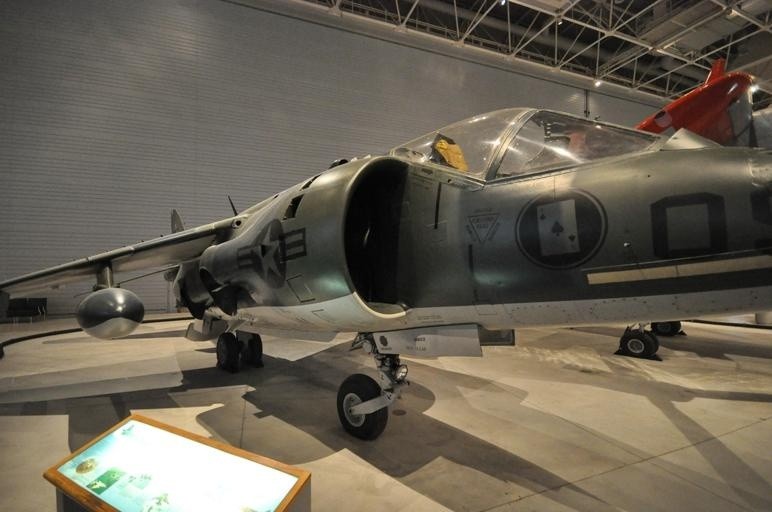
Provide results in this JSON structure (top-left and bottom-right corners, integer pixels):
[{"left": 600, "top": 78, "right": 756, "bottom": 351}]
[
  {"left": 0, "top": 54, "right": 772, "bottom": 438},
  {"left": 590, "top": 56, "right": 772, "bottom": 365}
]
